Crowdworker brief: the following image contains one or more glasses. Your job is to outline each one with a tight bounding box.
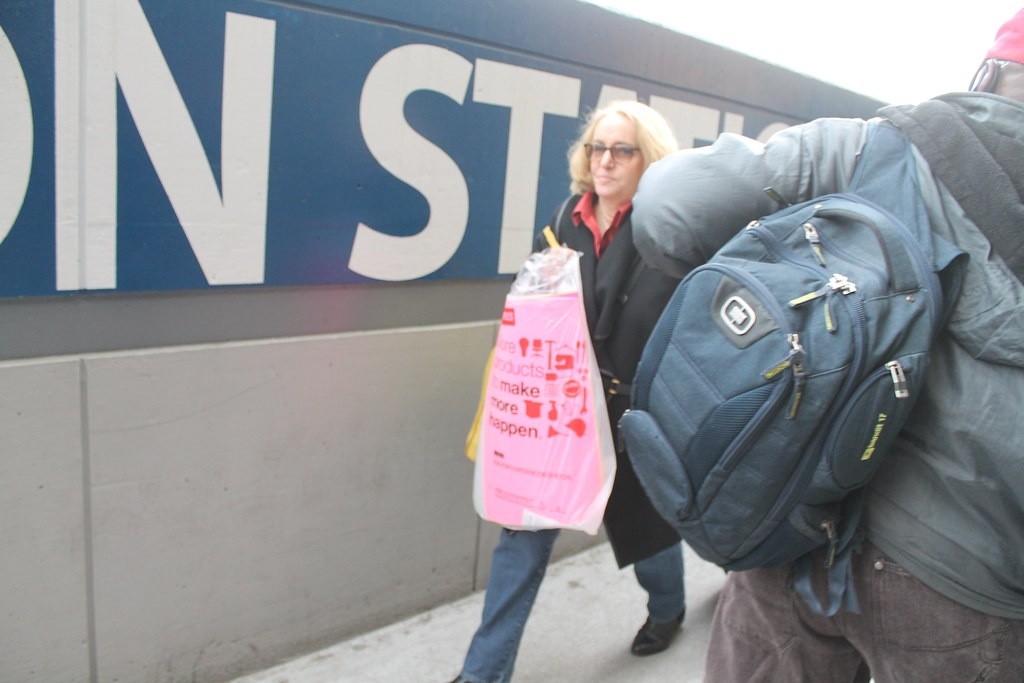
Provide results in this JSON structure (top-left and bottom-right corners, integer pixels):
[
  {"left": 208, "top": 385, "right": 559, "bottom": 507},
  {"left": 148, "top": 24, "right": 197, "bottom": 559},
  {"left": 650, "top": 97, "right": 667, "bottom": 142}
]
[{"left": 584, "top": 141, "right": 642, "bottom": 164}]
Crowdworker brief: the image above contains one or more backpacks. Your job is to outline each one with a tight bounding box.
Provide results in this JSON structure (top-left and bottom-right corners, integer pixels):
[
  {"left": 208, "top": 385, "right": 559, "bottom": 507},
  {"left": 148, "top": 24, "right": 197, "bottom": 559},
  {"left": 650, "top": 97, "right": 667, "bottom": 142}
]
[{"left": 617, "top": 118, "right": 966, "bottom": 576}]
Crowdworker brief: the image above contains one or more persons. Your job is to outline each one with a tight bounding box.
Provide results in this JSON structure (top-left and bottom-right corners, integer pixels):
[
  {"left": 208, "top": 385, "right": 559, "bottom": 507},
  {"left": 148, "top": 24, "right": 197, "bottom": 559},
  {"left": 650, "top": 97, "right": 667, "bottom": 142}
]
[
  {"left": 631, "top": 8, "right": 1024, "bottom": 682},
  {"left": 449, "top": 99, "right": 685, "bottom": 682}
]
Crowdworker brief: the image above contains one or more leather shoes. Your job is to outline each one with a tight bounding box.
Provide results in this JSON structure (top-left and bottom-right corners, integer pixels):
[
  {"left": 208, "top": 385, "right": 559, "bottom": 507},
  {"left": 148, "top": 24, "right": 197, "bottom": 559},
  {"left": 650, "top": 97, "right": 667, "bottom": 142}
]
[{"left": 631, "top": 609, "right": 689, "bottom": 656}]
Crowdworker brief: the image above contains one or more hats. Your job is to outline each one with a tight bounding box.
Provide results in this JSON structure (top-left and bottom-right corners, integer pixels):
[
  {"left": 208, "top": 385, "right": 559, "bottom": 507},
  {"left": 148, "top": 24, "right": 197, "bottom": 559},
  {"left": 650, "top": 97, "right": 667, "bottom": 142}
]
[{"left": 986, "top": 5, "right": 1024, "bottom": 64}]
